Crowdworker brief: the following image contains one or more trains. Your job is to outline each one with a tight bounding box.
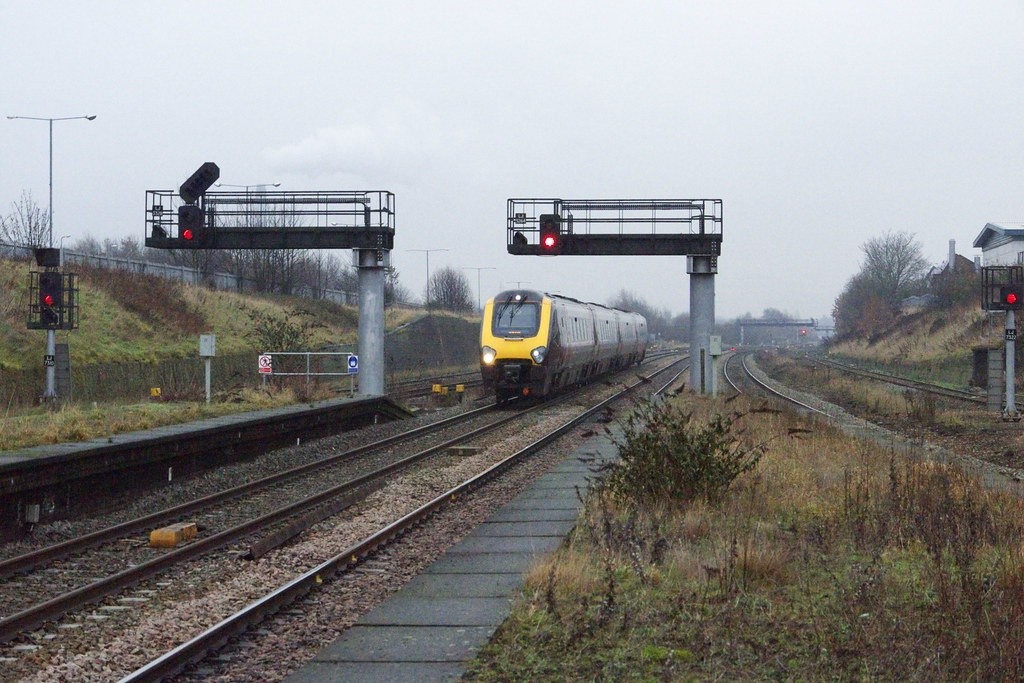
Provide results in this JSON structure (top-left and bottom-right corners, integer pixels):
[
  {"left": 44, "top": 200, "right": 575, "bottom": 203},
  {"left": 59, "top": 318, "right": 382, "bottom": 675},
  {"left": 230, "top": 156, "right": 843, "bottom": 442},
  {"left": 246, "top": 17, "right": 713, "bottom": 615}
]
[{"left": 479, "top": 285, "right": 648, "bottom": 408}]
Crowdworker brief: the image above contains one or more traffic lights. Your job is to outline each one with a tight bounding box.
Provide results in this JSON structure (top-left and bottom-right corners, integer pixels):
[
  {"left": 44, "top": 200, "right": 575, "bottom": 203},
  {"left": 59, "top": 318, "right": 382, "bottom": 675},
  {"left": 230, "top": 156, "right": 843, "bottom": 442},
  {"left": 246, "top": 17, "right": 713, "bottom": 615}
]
[
  {"left": 40, "top": 272, "right": 62, "bottom": 308},
  {"left": 178, "top": 205, "right": 202, "bottom": 244},
  {"left": 540, "top": 214, "right": 560, "bottom": 253},
  {"left": 999, "top": 286, "right": 1024, "bottom": 308}
]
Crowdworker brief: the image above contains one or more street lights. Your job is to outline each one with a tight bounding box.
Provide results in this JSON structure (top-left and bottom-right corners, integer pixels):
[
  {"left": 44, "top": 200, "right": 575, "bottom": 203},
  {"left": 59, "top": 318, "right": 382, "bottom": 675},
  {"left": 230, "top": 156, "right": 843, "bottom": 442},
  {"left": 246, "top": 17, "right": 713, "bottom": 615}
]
[
  {"left": 214, "top": 182, "right": 281, "bottom": 261},
  {"left": 7, "top": 114, "right": 97, "bottom": 398},
  {"left": 462, "top": 267, "right": 496, "bottom": 309},
  {"left": 407, "top": 249, "right": 450, "bottom": 306},
  {"left": 60, "top": 234, "right": 72, "bottom": 269}
]
